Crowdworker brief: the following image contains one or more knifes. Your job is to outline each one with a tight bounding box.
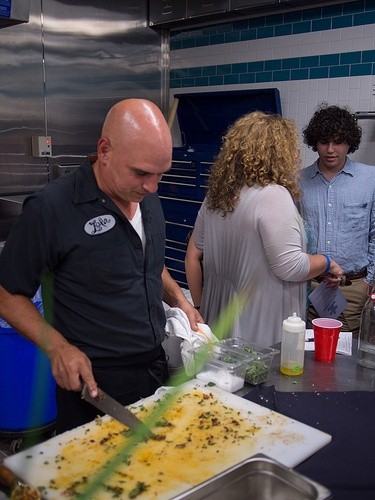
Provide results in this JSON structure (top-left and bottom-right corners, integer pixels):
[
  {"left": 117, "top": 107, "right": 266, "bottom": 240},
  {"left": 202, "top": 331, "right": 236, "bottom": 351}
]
[{"left": 80, "top": 377, "right": 153, "bottom": 437}]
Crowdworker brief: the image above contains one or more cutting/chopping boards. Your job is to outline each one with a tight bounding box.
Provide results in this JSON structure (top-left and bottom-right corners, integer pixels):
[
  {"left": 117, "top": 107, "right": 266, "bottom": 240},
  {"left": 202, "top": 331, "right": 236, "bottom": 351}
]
[{"left": 3, "top": 379, "right": 332, "bottom": 500}]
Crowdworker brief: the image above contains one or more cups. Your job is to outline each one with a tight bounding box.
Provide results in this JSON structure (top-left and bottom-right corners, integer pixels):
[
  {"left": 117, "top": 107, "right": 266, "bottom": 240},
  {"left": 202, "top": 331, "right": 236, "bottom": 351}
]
[{"left": 312, "top": 318, "right": 343, "bottom": 363}]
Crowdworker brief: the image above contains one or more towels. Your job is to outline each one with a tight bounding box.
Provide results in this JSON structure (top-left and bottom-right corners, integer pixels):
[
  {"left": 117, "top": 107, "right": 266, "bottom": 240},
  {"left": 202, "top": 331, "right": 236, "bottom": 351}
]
[{"left": 164, "top": 303, "right": 219, "bottom": 376}]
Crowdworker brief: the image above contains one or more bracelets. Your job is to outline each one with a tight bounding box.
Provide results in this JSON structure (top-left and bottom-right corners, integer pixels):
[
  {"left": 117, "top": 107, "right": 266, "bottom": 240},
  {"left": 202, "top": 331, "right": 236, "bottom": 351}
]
[{"left": 320, "top": 254, "right": 330, "bottom": 274}]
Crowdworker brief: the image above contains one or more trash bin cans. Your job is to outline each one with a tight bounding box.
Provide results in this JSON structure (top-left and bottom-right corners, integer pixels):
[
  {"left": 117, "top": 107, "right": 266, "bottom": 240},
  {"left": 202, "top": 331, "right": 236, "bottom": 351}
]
[{"left": 0, "top": 286, "right": 57, "bottom": 454}]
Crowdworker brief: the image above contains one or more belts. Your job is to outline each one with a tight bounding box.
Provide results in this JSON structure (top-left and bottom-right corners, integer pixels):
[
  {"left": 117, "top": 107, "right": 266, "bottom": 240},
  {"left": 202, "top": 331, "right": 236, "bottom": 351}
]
[{"left": 311, "top": 266, "right": 367, "bottom": 282}]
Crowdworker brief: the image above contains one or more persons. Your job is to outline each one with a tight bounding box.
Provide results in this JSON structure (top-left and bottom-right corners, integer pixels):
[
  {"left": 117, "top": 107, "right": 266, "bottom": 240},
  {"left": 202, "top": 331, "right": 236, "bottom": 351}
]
[
  {"left": 0, "top": 98, "right": 206, "bottom": 434},
  {"left": 295, "top": 105, "right": 375, "bottom": 330},
  {"left": 184, "top": 111, "right": 345, "bottom": 352}
]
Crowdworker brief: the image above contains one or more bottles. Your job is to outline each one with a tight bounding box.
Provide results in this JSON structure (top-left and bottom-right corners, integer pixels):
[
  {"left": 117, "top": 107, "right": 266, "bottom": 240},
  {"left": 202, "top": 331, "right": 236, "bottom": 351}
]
[
  {"left": 356, "top": 281, "right": 375, "bottom": 368},
  {"left": 280, "top": 312, "right": 306, "bottom": 375}
]
